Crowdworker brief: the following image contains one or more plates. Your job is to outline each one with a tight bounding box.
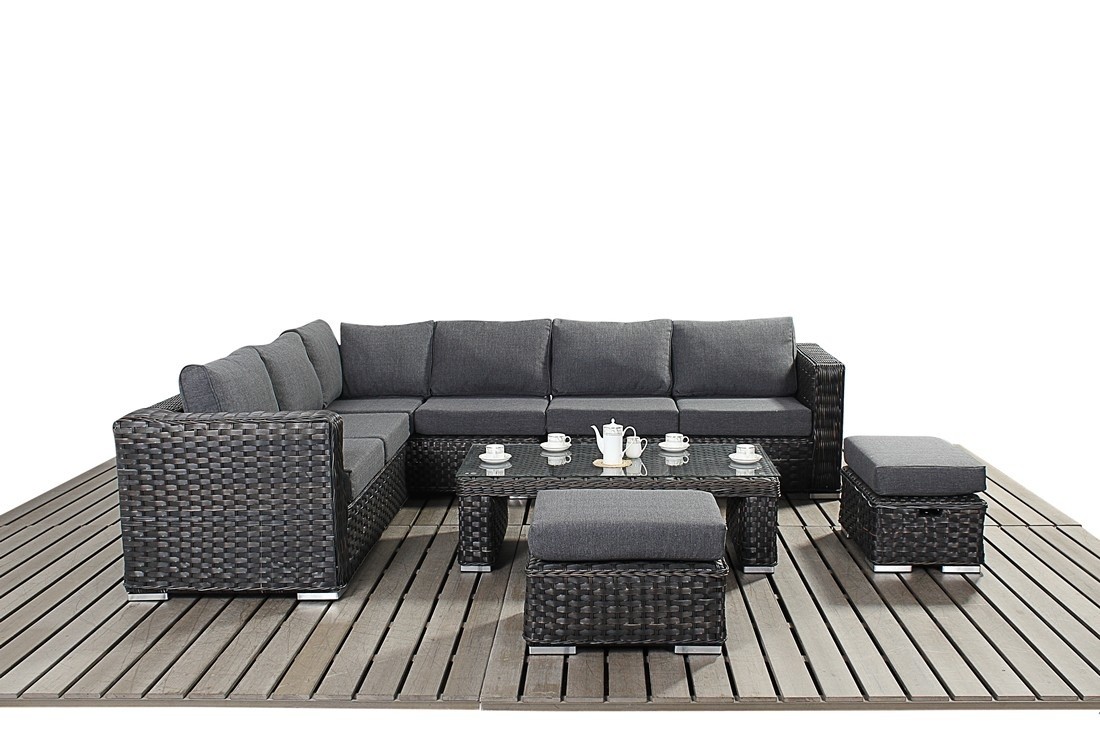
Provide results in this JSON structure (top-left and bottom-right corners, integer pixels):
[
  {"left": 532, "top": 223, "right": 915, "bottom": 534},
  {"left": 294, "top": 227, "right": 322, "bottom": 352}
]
[
  {"left": 540, "top": 442, "right": 573, "bottom": 452},
  {"left": 479, "top": 453, "right": 513, "bottom": 464},
  {"left": 657, "top": 442, "right": 690, "bottom": 452},
  {"left": 729, "top": 453, "right": 763, "bottom": 464}
]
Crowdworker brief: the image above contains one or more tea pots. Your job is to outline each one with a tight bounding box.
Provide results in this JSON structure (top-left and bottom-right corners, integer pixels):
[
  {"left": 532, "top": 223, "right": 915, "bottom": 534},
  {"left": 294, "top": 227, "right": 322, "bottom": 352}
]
[{"left": 590, "top": 418, "right": 636, "bottom": 465}]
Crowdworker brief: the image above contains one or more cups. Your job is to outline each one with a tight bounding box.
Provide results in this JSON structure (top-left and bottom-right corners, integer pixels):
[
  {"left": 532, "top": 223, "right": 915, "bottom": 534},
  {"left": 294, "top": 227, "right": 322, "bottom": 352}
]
[
  {"left": 546, "top": 433, "right": 571, "bottom": 448},
  {"left": 626, "top": 436, "right": 647, "bottom": 458},
  {"left": 735, "top": 443, "right": 756, "bottom": 459},
  {"left": 486, "top": 444, "right": 504, "bottom": 459},
  {"left": 665, "top": 433, "right": 690, "bottom": 449}
]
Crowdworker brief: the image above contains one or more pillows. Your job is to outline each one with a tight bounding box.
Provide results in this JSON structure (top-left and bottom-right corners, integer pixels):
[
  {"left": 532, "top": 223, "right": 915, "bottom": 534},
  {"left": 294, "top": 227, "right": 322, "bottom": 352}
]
[
  {"left": 431, "top": 319, "right": 555, "bottom": 400},
  {"left": 243, "top": 333, "right": 327, "bottom": 411},
  {"left": 670, "top": 319, "right": 803, "bottom": 397},
  {"left": 549, "top": 319, "right": 676, "bottom": 400},
  {"left": 175, "top": 342, "right": 275, "bottom": 412},
  {"left": 338, "top": 319, "right": 434, "bottom": 398},
  {"left": 280, "top": 318, "right": 344, "bottom": 407}
]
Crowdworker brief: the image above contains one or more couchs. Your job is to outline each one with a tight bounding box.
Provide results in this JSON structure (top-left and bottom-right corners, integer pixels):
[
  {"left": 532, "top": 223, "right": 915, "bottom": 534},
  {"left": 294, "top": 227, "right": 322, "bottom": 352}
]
[{"left": 111, "top": 341, "right": 849, "bottom": 609}]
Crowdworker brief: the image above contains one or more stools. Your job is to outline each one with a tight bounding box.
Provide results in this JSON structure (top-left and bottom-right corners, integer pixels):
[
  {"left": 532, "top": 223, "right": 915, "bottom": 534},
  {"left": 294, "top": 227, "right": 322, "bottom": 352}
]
[
  {"left": 526, "top": 489, "right": 731, "bottom": 657},
  {"left": 836, "top": 434, "right": 987, "bottom": 576}
]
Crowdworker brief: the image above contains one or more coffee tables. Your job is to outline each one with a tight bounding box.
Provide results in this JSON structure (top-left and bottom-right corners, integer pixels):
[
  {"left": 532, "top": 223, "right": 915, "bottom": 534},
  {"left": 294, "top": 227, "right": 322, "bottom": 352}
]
[{"left": 451, "top": 440, "right": 788, "bottom": 576}]
